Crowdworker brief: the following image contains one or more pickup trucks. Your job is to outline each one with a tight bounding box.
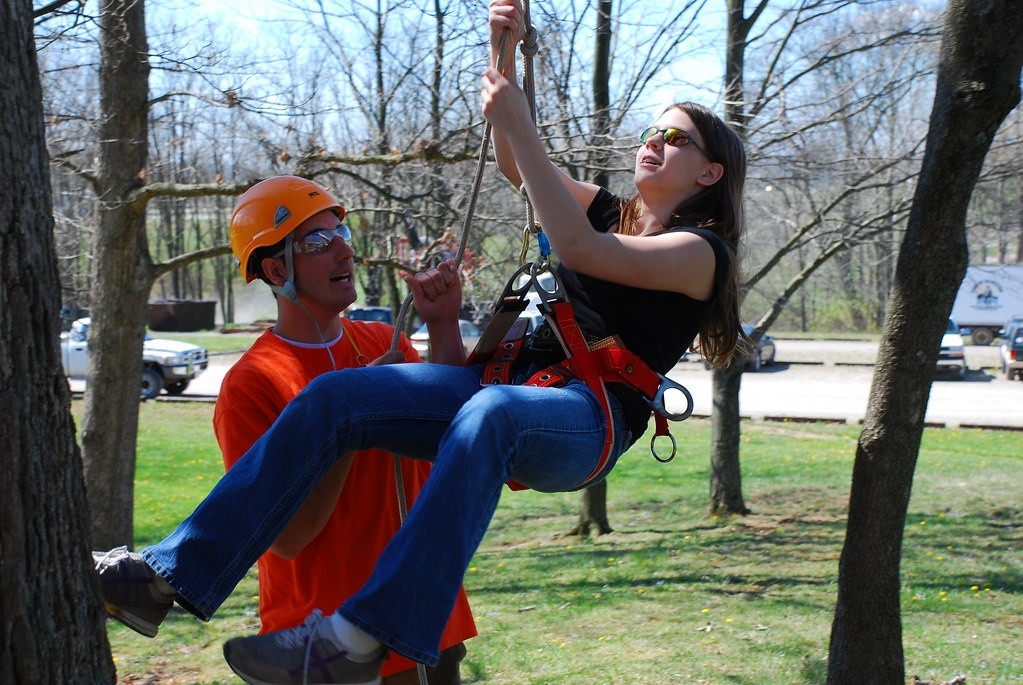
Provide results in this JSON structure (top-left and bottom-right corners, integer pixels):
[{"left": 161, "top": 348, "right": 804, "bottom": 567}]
[{"left": 60, "top": 317, "right": 208, "bottom": 399}]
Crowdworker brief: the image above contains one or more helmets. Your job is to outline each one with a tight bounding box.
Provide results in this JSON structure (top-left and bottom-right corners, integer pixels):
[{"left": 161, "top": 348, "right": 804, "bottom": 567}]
[{"left": 228, "top": 174, "right": 347, "bottom": 284}]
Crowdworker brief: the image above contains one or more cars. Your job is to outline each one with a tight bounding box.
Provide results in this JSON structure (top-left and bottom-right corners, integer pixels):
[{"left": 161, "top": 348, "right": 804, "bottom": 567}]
[
  {"left": 346, "top": 306, "right": 395, "bottom": 328},
  {"left": 934, "top": 316, "right": 972, "bottom": 380},
  {"left": 999, "top": 318, "right": 1023, "bottom": 380},
  {"left": 704, "top": 325, "right": 776, "bottom": 373},
  {"left": 409, "top": 320, "right": 482, "bottom": 362}
]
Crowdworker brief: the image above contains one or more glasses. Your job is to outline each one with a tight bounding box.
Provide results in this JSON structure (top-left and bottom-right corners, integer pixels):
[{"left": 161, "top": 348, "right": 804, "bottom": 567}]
[
  {"left": 269, "top": 223, "right": 352, "bottom": 259},
  {"left": 639, "top": 126, "right": 713, "bottom": 162}
]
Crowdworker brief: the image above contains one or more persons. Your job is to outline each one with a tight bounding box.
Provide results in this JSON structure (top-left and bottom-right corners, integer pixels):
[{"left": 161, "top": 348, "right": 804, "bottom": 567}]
[
  {"left": 212, "top": 175, "right": 478, "bottom": 685},
  {"left": 90, "top": 0, "right": 760, "bottom": 685}
]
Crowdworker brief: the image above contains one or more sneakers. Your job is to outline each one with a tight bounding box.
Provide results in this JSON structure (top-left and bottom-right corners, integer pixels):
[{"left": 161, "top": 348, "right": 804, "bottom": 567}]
[
  {"left": 223, "top": 608, "right": 386, "bottom": 685},
  {"left": 92, "top": 545, "right": 179, "bottom": 638}
]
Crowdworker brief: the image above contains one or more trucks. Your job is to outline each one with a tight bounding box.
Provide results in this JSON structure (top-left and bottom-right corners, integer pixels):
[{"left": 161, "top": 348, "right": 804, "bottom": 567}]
[{"left": 945, "top": 263, "right": 1023, "bottom": 346}]
